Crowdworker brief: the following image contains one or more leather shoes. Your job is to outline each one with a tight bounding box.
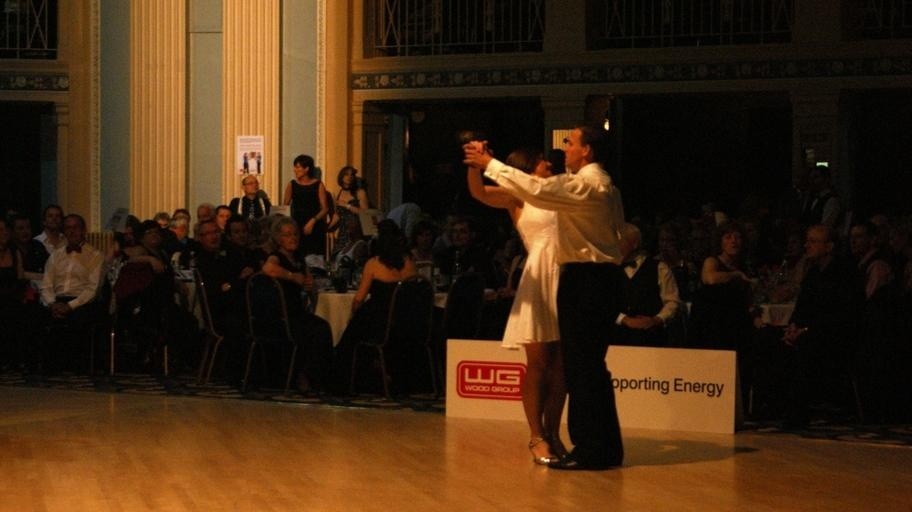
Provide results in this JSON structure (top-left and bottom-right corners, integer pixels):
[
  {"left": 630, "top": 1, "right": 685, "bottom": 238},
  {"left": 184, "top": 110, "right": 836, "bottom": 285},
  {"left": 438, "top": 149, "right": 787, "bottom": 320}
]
[
  {"left": 528, "top": 436, "right": 559, "bottom": 465},
  {"left": 544, "top": 433, "right": 569, "bottom": 457},
  {"left": 548, "top": 452, "right": 576, "bottom": 469}
]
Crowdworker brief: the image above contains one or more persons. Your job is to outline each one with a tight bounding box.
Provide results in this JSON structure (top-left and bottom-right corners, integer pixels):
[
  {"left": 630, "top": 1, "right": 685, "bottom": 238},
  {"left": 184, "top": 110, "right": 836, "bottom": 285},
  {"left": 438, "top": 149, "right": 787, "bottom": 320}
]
[
  {"left": 1, "top": 150, "right": 526, "bottom": 395},
  {"left": 609, "top": 197, "right": 911, "bottom": 437}
]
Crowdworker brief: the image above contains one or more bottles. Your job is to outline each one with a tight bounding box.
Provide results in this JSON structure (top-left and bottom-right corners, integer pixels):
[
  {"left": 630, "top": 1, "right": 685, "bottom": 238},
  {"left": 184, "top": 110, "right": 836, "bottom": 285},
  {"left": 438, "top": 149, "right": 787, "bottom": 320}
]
[
  {"left": 189, "top": 251, "right": 199, "bottom": 276},
  {"left": 352, "top": 255, "right": 361, "bottom": 291},
  {"left": 453, "top": 250, "right": 462, "bottom": 283},
  {"left": 326, "top": 262, "right": 333, "bottom": 282},
  {"left": 431, "top": 262, "right": 441, "bottom": 290}
]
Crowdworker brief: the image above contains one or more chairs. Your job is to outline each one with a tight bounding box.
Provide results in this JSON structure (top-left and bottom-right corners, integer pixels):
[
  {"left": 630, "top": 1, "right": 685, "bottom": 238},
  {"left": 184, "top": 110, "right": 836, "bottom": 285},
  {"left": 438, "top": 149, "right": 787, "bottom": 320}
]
[{"left": 1, "top": 208, "right": 912, "bottom": 428}]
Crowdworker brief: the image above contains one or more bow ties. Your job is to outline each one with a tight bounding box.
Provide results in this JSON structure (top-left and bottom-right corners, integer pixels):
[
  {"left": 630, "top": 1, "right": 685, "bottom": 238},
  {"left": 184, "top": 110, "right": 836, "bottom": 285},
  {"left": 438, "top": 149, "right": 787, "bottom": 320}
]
[
  {"left": 66, "top": 245, "right": 81, "bottom": 253},
  {"left": 623, "top": 259, "right": 637, "bottom": 268}
]
